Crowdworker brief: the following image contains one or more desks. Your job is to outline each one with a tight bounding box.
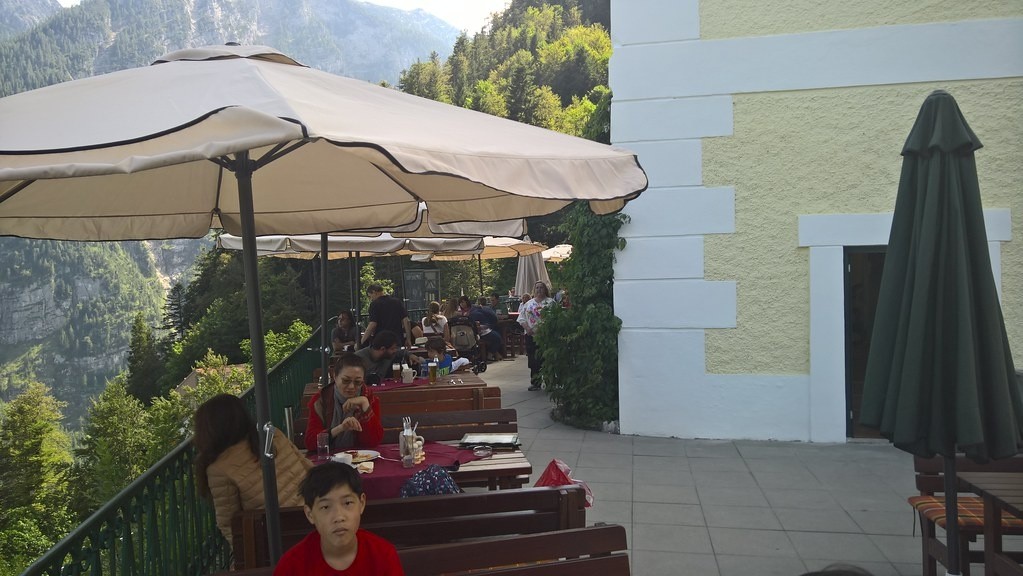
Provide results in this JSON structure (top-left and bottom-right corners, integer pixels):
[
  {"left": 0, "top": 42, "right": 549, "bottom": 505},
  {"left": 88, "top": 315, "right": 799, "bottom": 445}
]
[
  {"left": 303, "top": 372, "right": 487, "bottom": 394},
  {"left": 305, "top": 441, "right": 533, "bottom": 499},
  {"left": 955, "top": 471, "right": 1023, "bottom": 576}
]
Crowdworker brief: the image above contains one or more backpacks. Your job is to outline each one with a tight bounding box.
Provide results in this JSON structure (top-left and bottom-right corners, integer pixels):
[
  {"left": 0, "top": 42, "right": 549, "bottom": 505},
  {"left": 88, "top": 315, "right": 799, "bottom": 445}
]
[{"left": 402, "top": 464, "right": 462, "bottom": 498}]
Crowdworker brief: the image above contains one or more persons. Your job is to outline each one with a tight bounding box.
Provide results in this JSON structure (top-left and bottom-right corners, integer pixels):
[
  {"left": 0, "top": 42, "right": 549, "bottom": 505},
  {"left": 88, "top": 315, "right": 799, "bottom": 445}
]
[
  {"left": 331, "top": 309, "right": 367, "bottom": 379},
  {"left": 355, "top": 283, "right": 412, "bottom": 349},
  {"left": 403, "top": 301, "right": 450, "bottom": 359},
  {"left": 191, "top": 394, "right": 318, "bottom": 570},
  {"left": 490, "top": 293, "right": 506, "bottom": 316},
  {"left": 272, "top": 460, "right": 408, "bottom": 576},
  {"left": 506, "top": 287, "right": 520, "bottom": 311},
  {"left": 558, "top": 290, "right": 573, "bottom": 309},
  {"left": 517, "top": 280, "right": 558, "bottom": 390},
  {"left": 419, "top": 338, "right": 453, "bottom": 377},
  {"left": 479, "top": 298, "right": 498, "bottom": 324},
  {"left": 302, "top": 352, "right": 384, "bottom": 452},
  {"left": 351, "top": 328, "right": 429, "bottom": 376},
  {"left": 458, "top": 296, "right": 508, "bottom": 360}
]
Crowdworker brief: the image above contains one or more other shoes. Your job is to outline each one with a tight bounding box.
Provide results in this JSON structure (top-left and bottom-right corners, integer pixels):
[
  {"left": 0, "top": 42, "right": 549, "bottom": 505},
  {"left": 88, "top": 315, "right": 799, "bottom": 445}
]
[{"left": 528, "top": 386, "right": 541, "bottom": 391}]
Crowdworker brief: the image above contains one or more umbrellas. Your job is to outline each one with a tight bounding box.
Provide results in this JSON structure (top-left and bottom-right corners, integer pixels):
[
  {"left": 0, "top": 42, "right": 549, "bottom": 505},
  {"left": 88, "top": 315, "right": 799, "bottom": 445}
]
[
  {"left": 858, "top": 87, "right": 1023, "bottom": 576},
  {"left": 514, "top": 234, "right": 553, "bottom": 301},
  {"left": 281, "top": 237, "right": 545, "bottom": 316},
  {"left": 0, "top": 38, "right": 648, "bottom": 570},
  {"left": 213, "top": 225, "right": 533, "bottom": 455},
  {"left": 252, "top": 240, "right": 494, "bottom": 368}
]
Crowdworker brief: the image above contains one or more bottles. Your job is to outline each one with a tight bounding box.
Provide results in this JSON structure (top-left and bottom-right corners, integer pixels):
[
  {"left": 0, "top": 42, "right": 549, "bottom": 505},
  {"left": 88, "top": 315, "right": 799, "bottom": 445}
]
[{"left": 433, "top": 355, "right": 440, "bottom": 380}]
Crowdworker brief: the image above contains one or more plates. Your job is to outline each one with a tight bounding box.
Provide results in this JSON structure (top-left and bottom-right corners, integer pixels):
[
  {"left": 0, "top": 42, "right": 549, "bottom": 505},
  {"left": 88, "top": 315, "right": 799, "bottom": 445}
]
[{"left": 334, "top": 450, "right": 380, "bottom": 463}]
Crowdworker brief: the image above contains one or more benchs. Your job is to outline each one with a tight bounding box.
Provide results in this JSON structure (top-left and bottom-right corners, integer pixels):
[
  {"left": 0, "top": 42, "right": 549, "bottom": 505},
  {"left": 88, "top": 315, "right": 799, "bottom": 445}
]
[
  {"left": 912, "top": 448, "right": 1023, "bottom": 576},
  {"left": 229, "top": 316, "right": 632, "bottom": 576}
]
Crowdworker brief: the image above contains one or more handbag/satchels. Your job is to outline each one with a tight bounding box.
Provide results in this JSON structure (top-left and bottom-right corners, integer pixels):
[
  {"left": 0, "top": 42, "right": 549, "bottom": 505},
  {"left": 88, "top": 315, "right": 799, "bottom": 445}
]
[{"left": 445, "top": 341, "right": 458, "bottom": 356}]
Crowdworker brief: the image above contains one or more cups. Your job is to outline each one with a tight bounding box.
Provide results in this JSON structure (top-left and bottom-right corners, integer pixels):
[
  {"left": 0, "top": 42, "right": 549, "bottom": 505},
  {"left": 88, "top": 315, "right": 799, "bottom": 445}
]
[
  {"left": 402, "top": 368, "right": 417, "bottom": 383},
  {"left": 399, "top": 429, "right": 426, "bottom": 468},
  {"left": 336, "top": 454, "right": 352, "bottom": 465},
  {"left": 392, "top": 363, "right": 401, "bottom": 383},
  {"left": 428, "top": 363, "right": 437, "bottom": 384},
  {"left": 316, "top": 433, "right": 330, "bottom": 460}
]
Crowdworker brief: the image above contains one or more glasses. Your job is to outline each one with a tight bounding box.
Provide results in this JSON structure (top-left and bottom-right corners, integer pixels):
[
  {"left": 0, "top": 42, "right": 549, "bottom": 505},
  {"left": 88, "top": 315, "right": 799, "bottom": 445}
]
[
  {"left": 337, "top": 317, "right": 349, "bottom": 320},
  {"left": 336, "top": 371, "right": 364, "bottom": 386}
]
